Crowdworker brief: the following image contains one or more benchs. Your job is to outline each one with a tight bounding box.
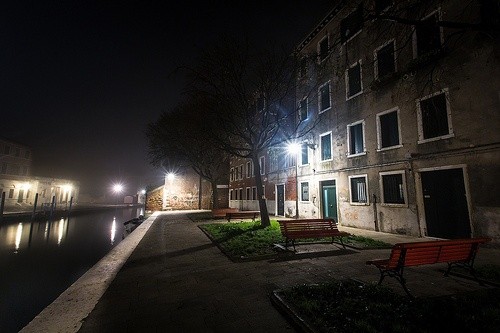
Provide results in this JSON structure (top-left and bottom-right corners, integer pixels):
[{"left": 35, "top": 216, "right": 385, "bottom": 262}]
[
  {"left": 365, "top": 236, "right": 492, "bottom": 302},
  {"left": 277, "top": 218, "right": 349, "bottom": 255},
  {"left": 224, "top": 212, "right": 259, "bottom": 223},
  {"left": 212, "top": 208, "right": 239, "bottom": 220}
]
